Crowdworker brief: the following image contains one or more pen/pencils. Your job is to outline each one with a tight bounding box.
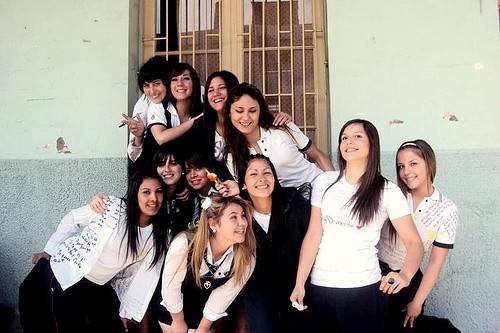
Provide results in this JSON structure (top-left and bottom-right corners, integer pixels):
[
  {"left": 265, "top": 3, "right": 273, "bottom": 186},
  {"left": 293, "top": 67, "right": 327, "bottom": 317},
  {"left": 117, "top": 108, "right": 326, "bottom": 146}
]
[{"left": 119, "top": 112, "right": 142, "bottom": 128}]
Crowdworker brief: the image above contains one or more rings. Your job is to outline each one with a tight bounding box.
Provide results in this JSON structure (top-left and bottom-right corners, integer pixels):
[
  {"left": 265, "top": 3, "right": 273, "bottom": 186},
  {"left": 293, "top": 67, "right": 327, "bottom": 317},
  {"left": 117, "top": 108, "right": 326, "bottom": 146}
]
[{"left": 388, "top": 278, "right": 395, "bottom": 284}]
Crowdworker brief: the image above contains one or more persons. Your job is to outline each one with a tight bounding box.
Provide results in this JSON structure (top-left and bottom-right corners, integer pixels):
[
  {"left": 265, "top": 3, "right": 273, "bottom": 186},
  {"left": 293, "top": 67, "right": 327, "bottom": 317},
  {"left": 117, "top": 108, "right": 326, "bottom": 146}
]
[
  {"left": 184, "top": 160, "right": 218, "bottom": 219},
  {"left": 119, "top": 55, "right": 178, "bottom": 162},
  {"left": 237, "top": 154, "right": 312, "bottom": 331},
  {"left": 141, "top": 63, "right": 201, "bottom": 169},
  {"left": 192, "top": 71, "right": 293, "bottom": 159},
  {"left": 204, "top": 83, "right": 333, "bottom": 196},
  {"left": 90, "top": 148, "right": 193, "bottom": 242},
  {"left": 158, "top": 196, "right": 257, "bottom": 332},
  {"left": 16, "top": 171, "right": 167, "bottom": 332},
  {"left": 289, "top": 119, "right": 461, "bottom": 333},
  {"left": 377, "top": 140, "right": 459, "bottom": 328}
]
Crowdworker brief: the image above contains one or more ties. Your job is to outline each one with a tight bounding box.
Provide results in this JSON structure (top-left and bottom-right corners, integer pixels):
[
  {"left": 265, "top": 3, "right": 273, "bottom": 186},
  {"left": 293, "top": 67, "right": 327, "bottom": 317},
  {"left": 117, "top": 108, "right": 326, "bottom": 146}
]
[{"left": 203, "top": 248, "right": 230, "bottom": 278}]
